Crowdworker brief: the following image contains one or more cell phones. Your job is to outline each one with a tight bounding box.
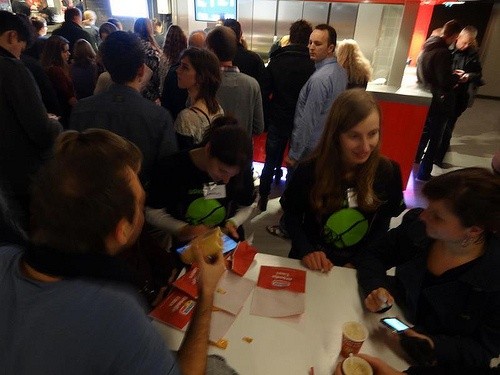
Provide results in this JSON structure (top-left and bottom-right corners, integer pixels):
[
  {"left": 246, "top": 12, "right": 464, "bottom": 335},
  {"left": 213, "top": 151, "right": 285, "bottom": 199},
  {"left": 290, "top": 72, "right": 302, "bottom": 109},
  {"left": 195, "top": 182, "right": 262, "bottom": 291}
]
[{"left": 380, "top": 317, "right": 411, "bottom": 335}]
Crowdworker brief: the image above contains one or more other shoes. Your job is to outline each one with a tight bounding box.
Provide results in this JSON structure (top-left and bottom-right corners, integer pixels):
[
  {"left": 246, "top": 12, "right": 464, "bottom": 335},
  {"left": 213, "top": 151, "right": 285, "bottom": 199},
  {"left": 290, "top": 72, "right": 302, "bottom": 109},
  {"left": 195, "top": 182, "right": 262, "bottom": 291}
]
[
  {"left": 415, "top": 173, "right": 433, "bottom": 183},
  {"left": 434, "top": 160, "right": 450, "bottom": 168},
  {"left": 257, "top": 198, "right": 268, "bottom": 212},
  {"left": 274, "top": 169, "right": 283, "bottom": 181},
  {"left": 415, "top": 158, "right": 421, "bottom": 164}
]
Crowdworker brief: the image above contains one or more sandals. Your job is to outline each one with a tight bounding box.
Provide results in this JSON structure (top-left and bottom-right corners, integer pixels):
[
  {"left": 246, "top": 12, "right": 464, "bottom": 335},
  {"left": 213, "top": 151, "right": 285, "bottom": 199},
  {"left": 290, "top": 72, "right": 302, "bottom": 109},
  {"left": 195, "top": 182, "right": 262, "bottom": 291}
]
[{"left": 266, "top": 224, "right": 291, "bottom": 240}]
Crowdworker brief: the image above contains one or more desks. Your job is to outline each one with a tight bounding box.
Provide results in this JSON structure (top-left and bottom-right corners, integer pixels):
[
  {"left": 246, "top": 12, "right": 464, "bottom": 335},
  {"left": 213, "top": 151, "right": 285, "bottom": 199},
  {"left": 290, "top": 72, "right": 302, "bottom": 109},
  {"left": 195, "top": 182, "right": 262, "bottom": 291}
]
[{"left": 149, "top": 252, "right": 412, "bottom": 375}]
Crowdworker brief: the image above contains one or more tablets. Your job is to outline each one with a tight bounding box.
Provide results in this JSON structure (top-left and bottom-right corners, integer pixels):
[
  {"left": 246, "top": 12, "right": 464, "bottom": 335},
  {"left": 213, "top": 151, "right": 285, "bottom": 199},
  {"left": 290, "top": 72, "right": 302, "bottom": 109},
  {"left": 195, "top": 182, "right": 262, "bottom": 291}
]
[{"left": 176, "top": 230, "right": 238, "bottom": 265}]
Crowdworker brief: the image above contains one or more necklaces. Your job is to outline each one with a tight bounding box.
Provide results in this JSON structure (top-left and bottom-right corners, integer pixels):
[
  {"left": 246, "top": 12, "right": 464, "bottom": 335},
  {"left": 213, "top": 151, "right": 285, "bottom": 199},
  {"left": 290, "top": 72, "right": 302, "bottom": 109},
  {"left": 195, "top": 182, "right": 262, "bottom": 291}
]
[{"left": 438, "top": 242, "right": 475, "bottom": 268}]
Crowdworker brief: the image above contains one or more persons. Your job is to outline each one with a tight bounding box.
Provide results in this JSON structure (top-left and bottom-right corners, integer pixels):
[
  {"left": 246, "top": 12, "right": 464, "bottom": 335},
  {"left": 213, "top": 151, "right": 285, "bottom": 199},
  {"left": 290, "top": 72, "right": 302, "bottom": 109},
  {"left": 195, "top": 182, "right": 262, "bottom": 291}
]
[
  {"left": 356, "top": 167, "right": 500, "bottom": 375},
  {"left": 288, "top": 88, "right": 407, "bottom": 273},
  {"left": 491, "top": 147, "right": 500, "bottom": 177},
  {"left": 414, "top": 20, "right": 485, "bottom": 181},
  {"left": 0, "top": 128, "right": 226, "bottom": 375},
  {"left": 0, "top": 0, "right": 373, "bottom": 310}
]
